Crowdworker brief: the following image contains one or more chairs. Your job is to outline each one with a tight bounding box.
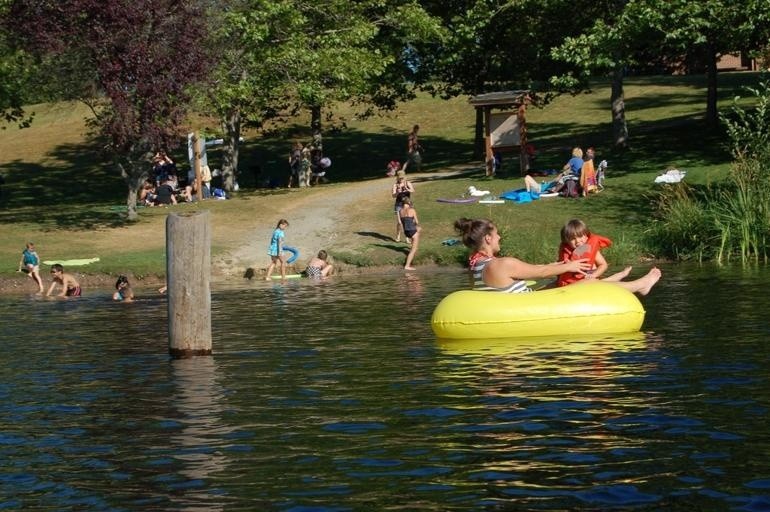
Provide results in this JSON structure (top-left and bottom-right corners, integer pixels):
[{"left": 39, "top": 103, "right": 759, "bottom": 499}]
[{"left": 563, "top": 159, "right": 608, "bottom": 196}]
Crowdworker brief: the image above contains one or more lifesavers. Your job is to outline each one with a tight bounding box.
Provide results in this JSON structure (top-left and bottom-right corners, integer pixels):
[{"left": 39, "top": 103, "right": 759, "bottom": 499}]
[{"left": 431, "top": 278, "right": 645, "bottom": 340}]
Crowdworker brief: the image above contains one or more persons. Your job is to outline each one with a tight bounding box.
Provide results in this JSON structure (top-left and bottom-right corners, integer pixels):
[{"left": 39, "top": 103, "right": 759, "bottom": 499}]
[
  {"left": 118, "top": 286, "right": 135, "bottom": 302},
  {"left": 563, "top": 146, "right": 585, "bottom": 177},
  {"left": 398, "top": 196, "right": 420, "bottom": 271},
  {"left": 45, "top": 263, "right": 82, "bottom": 297},
  {"left": 308, "top": 139, "right": 326, "bottom": 184},
  {"left": 400, "top": 124, "right": 426, "bottom": 173},
  {"left": 305, "top": 249, "right": 333, "bottom": 278},
  {"left": 524, "top": 173, "right": 565, "bottom": 193},
  {"left": 112, "top": 276, "right": 129, "bottom": 300},
  {"left": 391, "top": 170, "right": 415, "bottom": 244},
  {"left": 287, "top": 141, "right": 305, "bottom": 188},
  {"left": 16, "top": 242, "right": 44, "bottom": 293},
  {"left": 140, "top": 148, "right": 226, "bottom": 206},
  {"left": 263, "top": 219, "right": 290, "bottom": 280},
  {"left": 557, "top": 218, "right": 612, "bottom": 286},
  {"left": 453, "top": 216, "right": 662, "bottom": 296}
]
